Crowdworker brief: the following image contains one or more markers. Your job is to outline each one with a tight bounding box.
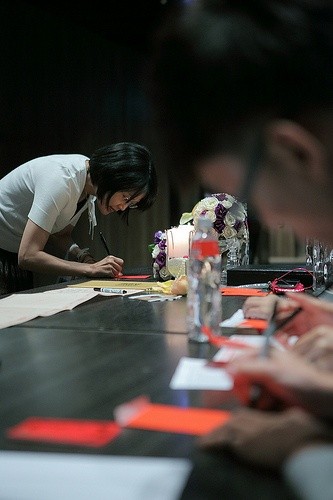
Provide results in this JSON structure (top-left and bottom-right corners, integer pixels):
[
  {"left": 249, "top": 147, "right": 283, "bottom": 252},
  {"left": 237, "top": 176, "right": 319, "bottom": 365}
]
[{"left": 94, "top": 287, "right": 127, "bottom": 294}]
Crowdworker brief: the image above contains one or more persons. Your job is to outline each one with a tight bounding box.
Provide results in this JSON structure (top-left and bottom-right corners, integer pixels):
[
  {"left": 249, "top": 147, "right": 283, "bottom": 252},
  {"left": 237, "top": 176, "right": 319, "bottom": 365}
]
[
  {"left": 0, "top": 142, "right": 160, "bottom": 306},
  {"left": 169, "top": 20, "right": 333, "bottom": 498},
  {"left": 265, "top": 283, "right": 333, "bottom": 379}
]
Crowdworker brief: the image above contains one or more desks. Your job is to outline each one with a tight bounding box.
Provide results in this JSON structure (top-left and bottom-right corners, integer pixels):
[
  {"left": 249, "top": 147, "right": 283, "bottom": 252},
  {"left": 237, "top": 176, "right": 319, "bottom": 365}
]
[{"left": 0, "top": 277, "right": 333, "bottom": 500}]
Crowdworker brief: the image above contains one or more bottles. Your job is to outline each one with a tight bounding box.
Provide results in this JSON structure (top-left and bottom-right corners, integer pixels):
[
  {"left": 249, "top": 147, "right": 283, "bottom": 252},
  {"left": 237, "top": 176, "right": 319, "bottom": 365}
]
[{"left": 185, "top": 214, "right": 222, "bottom": 342}]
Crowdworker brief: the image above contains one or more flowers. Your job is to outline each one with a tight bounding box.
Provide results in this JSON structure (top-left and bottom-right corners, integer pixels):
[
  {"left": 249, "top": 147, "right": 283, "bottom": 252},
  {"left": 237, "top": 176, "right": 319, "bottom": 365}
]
[
  {"left": 180, "top": 193, "right": 249, "bottom": 240},
  {"left": 152, "top": 230, "right": 170, "bottom": 282}
]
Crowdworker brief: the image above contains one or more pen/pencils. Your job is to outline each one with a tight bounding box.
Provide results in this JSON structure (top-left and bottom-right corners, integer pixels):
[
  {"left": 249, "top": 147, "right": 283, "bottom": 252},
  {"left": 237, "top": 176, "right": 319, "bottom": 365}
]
[
  {"left": 247, "top": 300, "right": 279, "bottom": 408},
  {"left": 99, "top": 231, "right": 120, "bottom": 278},
  {"left": 273, "top": 305, "right": 303, "bottom": 334}
]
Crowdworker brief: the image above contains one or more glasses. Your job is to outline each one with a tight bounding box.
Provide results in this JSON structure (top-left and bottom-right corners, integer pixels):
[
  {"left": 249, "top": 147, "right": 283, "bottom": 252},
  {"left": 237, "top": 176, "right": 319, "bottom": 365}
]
[{"left": 120, "top": 191, "right": 139, "bottom": 211}]
